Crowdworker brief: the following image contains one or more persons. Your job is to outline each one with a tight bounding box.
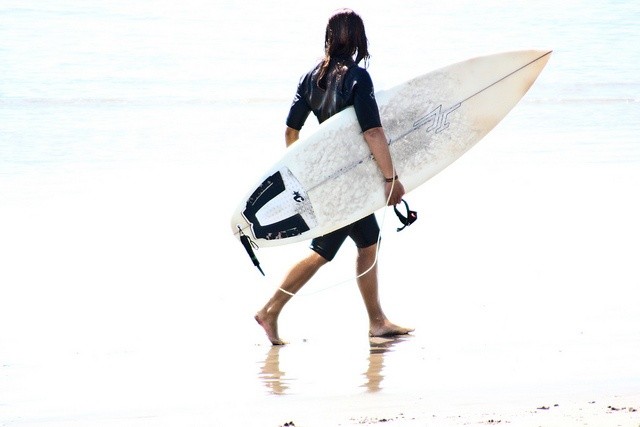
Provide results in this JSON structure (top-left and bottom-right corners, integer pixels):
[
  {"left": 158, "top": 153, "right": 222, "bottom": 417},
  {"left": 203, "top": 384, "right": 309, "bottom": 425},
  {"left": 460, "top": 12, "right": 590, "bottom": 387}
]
[{"left": 255, "top": 13, "right": 415, "bottom": 346}]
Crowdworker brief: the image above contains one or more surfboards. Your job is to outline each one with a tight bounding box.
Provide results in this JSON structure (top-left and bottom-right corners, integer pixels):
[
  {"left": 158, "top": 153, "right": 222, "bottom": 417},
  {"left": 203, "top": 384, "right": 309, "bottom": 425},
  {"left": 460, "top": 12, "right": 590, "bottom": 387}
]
[{"left": 230, "top": 50, "right": 553, "bottom": 249}]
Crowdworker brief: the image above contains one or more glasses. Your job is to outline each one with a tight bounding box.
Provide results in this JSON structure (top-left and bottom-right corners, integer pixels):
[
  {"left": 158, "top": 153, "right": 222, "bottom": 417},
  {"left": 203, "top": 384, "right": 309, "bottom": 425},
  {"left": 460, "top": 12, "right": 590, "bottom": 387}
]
[{"left": 393, "top": 199, "right": 417, "bottom": 232}]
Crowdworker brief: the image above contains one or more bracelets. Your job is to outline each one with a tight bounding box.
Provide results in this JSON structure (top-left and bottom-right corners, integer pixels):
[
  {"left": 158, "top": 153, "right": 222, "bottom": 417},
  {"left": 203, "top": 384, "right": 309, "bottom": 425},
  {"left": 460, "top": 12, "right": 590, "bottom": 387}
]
[{"left": 386, "top": 174, "right": 399, "bottom": 183}]
